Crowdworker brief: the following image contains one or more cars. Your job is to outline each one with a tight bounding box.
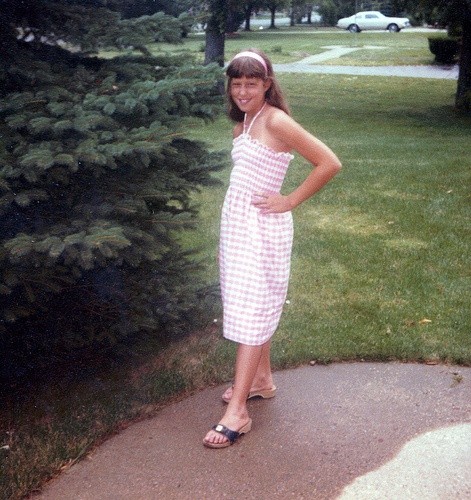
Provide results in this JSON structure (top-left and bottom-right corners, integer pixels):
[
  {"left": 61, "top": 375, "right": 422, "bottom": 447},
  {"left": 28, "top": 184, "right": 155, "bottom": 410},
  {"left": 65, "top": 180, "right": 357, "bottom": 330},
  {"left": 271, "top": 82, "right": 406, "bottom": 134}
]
[{"left": 336, "top": 11, "right": 410, "bottom": 33}]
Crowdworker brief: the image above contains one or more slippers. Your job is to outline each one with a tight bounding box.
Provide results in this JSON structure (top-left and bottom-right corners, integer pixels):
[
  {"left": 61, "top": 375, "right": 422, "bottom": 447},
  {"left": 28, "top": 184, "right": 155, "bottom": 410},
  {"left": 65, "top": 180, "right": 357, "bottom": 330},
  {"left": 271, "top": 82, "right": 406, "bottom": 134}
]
[
  {"left": 202, "top": 417, "right": 252, "bottom": 448},
  {"left": 222, "top": 382, "right": 276, "bottom": 403}
]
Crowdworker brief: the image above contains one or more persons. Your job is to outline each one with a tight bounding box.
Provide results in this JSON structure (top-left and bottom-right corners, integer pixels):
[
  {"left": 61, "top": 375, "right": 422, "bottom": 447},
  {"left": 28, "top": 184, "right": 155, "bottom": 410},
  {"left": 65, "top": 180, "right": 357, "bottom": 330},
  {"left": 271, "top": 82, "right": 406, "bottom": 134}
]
[{"left": 202, "top": 50, "right": 342, "bottom": 448}]
